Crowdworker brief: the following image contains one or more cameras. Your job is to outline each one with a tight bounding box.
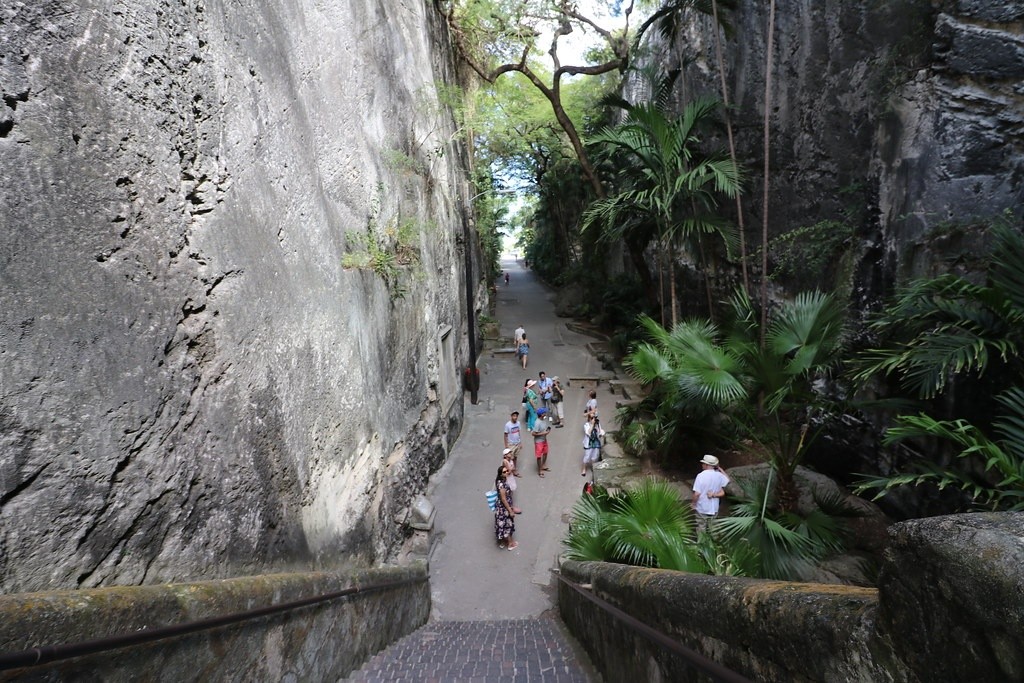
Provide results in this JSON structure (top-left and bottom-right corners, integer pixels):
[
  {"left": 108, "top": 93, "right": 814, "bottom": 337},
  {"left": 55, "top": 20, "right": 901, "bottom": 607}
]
[{"left": 594, "top": 418, "right": 599, "bottom": 424}]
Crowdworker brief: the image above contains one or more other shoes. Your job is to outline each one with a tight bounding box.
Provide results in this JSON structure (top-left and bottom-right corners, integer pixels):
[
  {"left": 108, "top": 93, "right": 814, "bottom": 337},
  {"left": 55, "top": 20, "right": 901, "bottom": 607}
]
[
  {"left": 524, "top": 420, "right": 528, "bottom": 425},
  {"left": 581, "top": 471, "right": 587, "bottom": 477}
]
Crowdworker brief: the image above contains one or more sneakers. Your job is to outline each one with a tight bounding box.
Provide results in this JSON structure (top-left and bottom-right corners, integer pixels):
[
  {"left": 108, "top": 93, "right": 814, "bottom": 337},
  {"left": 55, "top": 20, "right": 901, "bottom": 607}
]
[
  {"left": 548, "top": 416, "right": 553, "bottom": 422},
  {"left": 555, "top": 425, "right": 564, "bottom": 429},
  {"left": 526, "top": 427, "right": 531, "bottom": 432},
  {"left": 513, "top": 508, "right": 522, "bottom": 515},
  {"left": 552, "top": 421, "right": 560, "bottom": 425}
]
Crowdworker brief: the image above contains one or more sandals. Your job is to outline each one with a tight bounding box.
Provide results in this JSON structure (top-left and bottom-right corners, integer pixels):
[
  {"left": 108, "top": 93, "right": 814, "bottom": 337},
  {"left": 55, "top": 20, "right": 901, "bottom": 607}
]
[
  {"left": 538, "top": 473, "right": 545, "bottom": 478},
  {"left": 499, "top": 543, "right": 506, "bottom": 549},
  {"left": 513, "top": 472, "right": 522, "bottom": 478},
  {"left": 541, "top": 466, "right": 552, "bottom": 471},
  {"left": 507, "top": 541, "right": 519, "bottom": 551}
]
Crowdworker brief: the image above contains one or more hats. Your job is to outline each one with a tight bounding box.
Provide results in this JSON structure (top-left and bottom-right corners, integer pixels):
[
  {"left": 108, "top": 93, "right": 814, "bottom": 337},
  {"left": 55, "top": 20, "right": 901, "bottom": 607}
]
[
  {"left": 699, "top": 454, "right": 719, "bottom": 467},
  {"left": 551, "top": 375, "right": 561, "bottom": 382},
  {"left": 510, "top": 410, "right": 520, "bottom": 416},
  {"left": 502, "top": 449, "right": 514, "bottom": 456},
  {"left": 526, "top": 380, "right": 538, "bottom": 389},
  {"left": 536, "top": 408, "right": 547, "bottom": 416},
  {"left": 588, "top": 413, "right": 595, "bottom": 417}
]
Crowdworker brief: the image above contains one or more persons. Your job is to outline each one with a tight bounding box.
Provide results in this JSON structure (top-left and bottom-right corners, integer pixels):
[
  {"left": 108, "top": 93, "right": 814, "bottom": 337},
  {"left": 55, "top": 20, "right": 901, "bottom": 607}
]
[
  {"left": 514, "top": 324, "right": 530, "bottom": 370},
  {"left": 581, "top": 389, "right": 604, "bottom": 475},
  {"left": 504, "top": 273, "right": 509, "bottom": 285},
  {"left": 525, "top": 260, "right": 528, "bottom": 268},
  {"left": 494, "top": 371, "right": 565, "bottom": 551},
  {"left": 693, "top": 454, "right": 730, "bottom": 534}
]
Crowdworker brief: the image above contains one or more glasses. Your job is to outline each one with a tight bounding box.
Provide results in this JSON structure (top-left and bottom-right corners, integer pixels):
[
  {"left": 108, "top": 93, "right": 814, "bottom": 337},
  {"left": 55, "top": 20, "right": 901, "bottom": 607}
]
[
  {"left": 502, "top": 470, "right": 509, "bottom": 474},
  {"left": 590, "top": 417, "right": 594, "bottom": 420}
]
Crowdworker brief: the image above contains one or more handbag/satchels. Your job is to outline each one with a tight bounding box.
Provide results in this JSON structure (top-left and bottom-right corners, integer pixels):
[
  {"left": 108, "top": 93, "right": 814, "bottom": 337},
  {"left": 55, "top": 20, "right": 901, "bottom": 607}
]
[
  {"left": 485, "top": 479, "right": 500, "bottom": 512},
  {"left": 551, "top": 396, "right": 559, "bottom": 403},
  {"left": 522, "top": 395, "right": 527, "bottom": 409}
]
